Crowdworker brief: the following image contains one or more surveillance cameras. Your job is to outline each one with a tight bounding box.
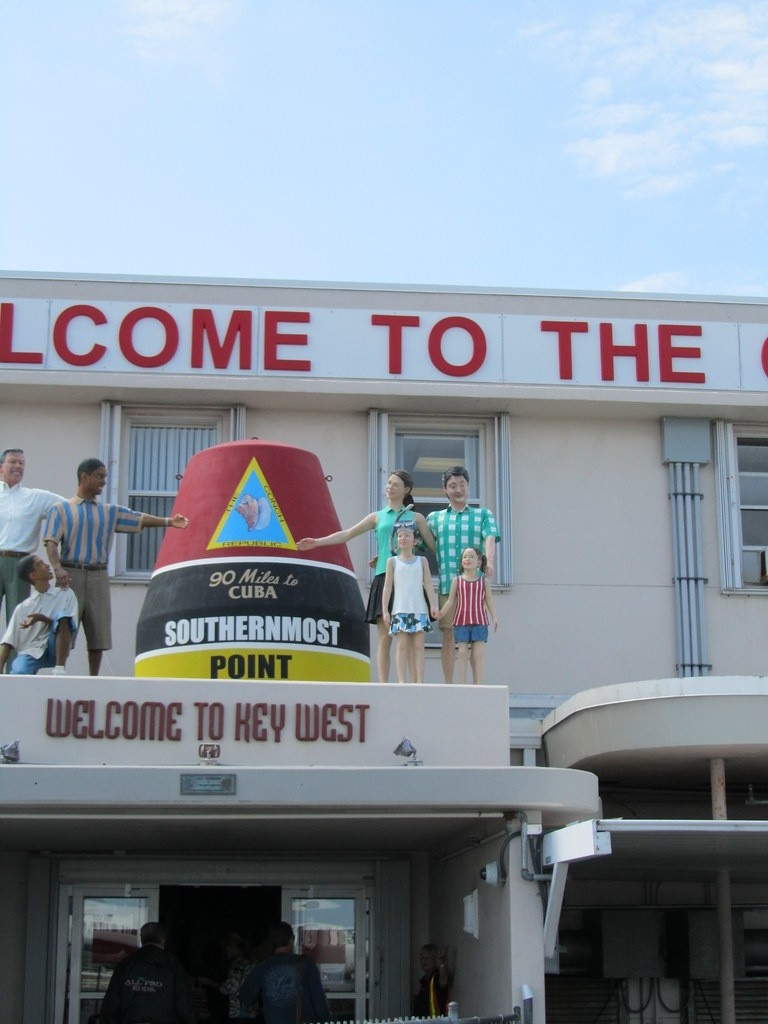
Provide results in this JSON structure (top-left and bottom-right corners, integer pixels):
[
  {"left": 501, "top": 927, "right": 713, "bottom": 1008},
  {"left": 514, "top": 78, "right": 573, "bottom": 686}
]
[{"left": 480, "top": 861, "right": 498, "bottom": 884}]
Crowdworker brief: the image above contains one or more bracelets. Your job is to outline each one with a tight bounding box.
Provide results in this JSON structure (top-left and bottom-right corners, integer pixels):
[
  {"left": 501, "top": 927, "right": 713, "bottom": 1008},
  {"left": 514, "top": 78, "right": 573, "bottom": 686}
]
[
  {"left": 166, "top": 517, "right": 169, "bottom": 527},
  {"left": 440, "top": 962, "right": 447, "bottom": 969}
]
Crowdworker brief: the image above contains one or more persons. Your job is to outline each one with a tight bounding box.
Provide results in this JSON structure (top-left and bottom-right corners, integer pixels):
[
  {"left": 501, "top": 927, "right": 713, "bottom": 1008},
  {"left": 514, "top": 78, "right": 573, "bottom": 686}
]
[
  {"left": 368, "top": 466, "right": 501, "bottom": 684},
  {"left": 0, "top": 555, "right": 77, "bottom": 676},
  {"left": 99, "top": 922, "right": 199, "bottom": 1024},
  {"left": 408, "top": 944, "right": 451, "bottom": 1018},
  {"left": 44, "top": 458, "right": 189, "bottom": 676},
  {"left": 381, "top": 521, "right": 439, "bottom": 683},
  {"left": 296, "top": 470, "right": 436, "bottom": 682},
  {"left": 240, "top": 923, "right": 332, "bottom": 1024},
  {"left": 159, "top": 935, "right": 210, "bottom": 1024},
  {"left": 436, "top": 548, "right": 499, "bottom": 685},
  {"left": 0, "top": 449, "right": 68, "bottom": 674},
  {"left": 195, "top": 933, "right": 259, "bottom": 1024}
]
